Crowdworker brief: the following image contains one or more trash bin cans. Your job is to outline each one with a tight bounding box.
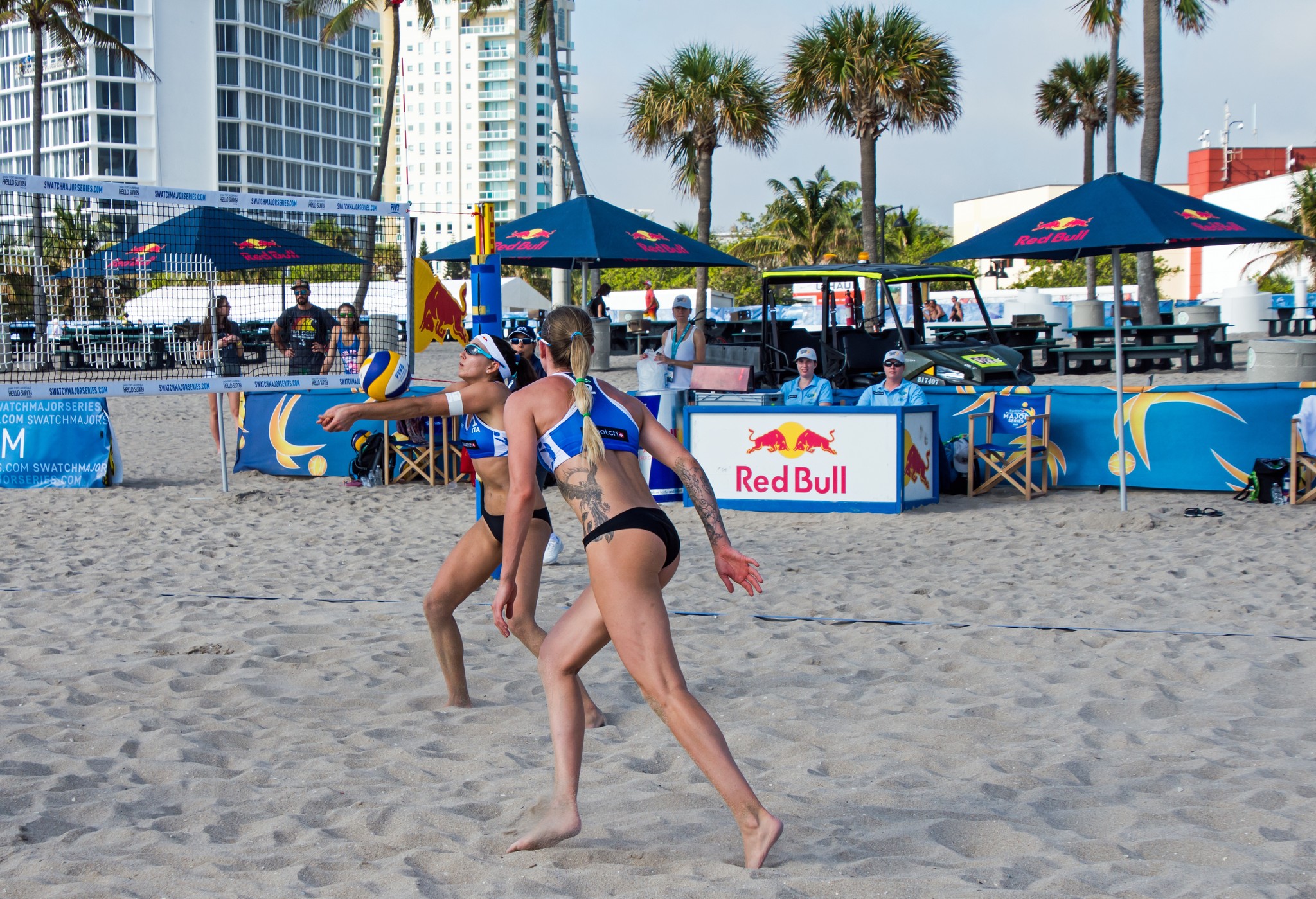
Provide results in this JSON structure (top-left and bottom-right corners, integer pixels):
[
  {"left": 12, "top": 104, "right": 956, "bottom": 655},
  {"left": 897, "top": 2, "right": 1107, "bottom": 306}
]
[
  {"left": 590, "top": 315, "right": 611, "bottom": 371},
  {"left": 1248, "top": 339, "right": 1316, "bottom": 383},
  {"left": 1071, "top": 299, "right": 1104, "bottom": 343},
  {"left": 368, "top": 314, "right": 400, "bottom": 351},
  {"left": 1173, "top": 305, "right": 1220, "bottom": 365}
]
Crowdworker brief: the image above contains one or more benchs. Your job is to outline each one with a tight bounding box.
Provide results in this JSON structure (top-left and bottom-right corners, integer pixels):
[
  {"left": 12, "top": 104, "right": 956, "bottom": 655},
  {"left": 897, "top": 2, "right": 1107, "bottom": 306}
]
[
  {"left": 731, "top": 330, "right": 766, "bottom": 345},
  {"left": 1260, "top": 318, "right": 1316, "bottom": 340},
  {"left": 1054, "top": 338, "right": 1245, "bottom": 373},
  {"left": 9, "top": 338, "right": 69, "bottom": 350},
  {"left": 90, "top": 339, "right": 148, "bottom": 369},
  {"left": 624, "top": 332, "right": 662, "bottom": 350},
  {"left": 51, "top": 349, "right": 161, "bottom": 372},
  {"left": 165, "top": 345, "right": 197, "bottom": 368},
  {"left": 10, "top": 345, "right": 30, "bottom": 361}
]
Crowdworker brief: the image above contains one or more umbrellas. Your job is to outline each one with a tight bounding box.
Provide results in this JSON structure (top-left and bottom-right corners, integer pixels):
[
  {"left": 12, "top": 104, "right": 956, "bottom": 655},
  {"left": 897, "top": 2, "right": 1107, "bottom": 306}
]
[
  {"left": 919, "top": 172, "right": 1316, "bottom": 511},
  {"left": 422, "top": 194, "right": 756, "bottom": 310},
  {"left": 42, "top": 206, "right": 374, "bottom": 491}
]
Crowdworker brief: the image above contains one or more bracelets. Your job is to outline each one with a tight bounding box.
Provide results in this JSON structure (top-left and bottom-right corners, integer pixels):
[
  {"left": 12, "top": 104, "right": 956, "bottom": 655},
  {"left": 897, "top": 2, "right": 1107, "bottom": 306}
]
[{"left": 236, "top": 340, "right": 241, "bottom": 346}]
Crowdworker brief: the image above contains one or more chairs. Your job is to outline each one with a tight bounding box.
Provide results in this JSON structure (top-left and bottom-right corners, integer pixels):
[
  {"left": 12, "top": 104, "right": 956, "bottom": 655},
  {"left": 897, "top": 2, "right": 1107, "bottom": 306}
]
[
  {"left": 378, "top": 408, "right": 481, "bottom": 489},
  {"left": 1289, "top": 394, "right": 1316, "bottom": 505},
  {"left": 966, "top": 393, "right": 1057, "bottom": 505},
  {"left": 1005, "top": 337, "right": 1074, "bottom": 374}
]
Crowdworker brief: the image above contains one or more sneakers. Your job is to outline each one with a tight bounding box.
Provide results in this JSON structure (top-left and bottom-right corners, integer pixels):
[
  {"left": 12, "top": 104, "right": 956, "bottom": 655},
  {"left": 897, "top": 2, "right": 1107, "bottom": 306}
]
[{"left": 543, "top": 535, "right": 563, "bottom": 564}]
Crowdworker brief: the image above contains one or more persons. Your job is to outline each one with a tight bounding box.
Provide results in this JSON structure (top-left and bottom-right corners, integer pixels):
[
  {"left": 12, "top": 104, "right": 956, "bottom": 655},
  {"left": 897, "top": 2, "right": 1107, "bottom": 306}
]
[
  {"left": 856, "top": 350, "right": 927, "bottom": 406},
  {"left": 639, "top": 295, "right": 706, "bottom": 405},
  {"left": 271, "top": 279, "right": 340, "bottom": 376},
  {"left": 319, "top": 302, "right": 371, "bottom": 375},
  {"left": 590, "top": 283, "right": 611, "bottom": 318},
  {"left": 315, "top": 333, "right": 608, "bottom": 729},
  {"left": 492, "top": 305, "right": 785, "bottom": 871},
  {"left": 197, "top": 295, "right": 244, "bottom": 455},
  {"left": 922, "top": 296, "right": 964, "bottom": 322},
  {"left": 642, "top": 280, "right": 658, "bottom": 321},
  {"left": 507, "top": 326, "right": 564, "bottom": 564},
  {"left": 781, "top": 347, "right": 833, "bottom": 406},
  {"left": 51, "top": 313, "right": 146, "bottom": 337}
]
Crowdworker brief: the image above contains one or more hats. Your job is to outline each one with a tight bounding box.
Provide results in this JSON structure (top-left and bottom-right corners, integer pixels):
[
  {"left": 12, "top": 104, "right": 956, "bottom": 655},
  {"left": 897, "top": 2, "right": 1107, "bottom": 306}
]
[
  {"left": 508, "top": 325, "right": 536, "bottom": 340},
  {"left": 642, "top": 280, "right": 652, "bottom": 287},
  {"left": 65, "top": 322, "right": 67, "bottom": 324},
  {"left": 672, "top": 294, "right": 691, "bottom": 309},
  {"left": 290, "top": 279, "right": 310, "bottom": 290},
  {"left": 794, "top": 347, "right": 817, "bottom": 362},
  {"left": 474, "top": 333, "right": 518, "bottom": 381},
  {"left": 53, "top": 318, "right": 58, "bottom": 323},
  {"left": 883, "top": 350, "right": 905, "bottom": 364},
  {"left": 953, "top": 438, "right": 969, "bottom": 473}
]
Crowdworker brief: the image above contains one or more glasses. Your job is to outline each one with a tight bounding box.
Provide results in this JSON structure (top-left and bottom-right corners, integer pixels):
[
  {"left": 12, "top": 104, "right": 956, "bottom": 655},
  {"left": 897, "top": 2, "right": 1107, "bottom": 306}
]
[
  {"left": 464, "top": 344, "right": 507, "bottom": 368},
  {"left": 338, "top": 313, "right": 355, "bottom": 318},
  {"left": 645, "top": 284, "right": 649, "bottom": 285},
  {"left": 294, "top": 290, "right": 309, "bottom": 296},
  {"left": 219, "top": 302, "right": 230, "bottom": 308},
  {"left": 884, "top": 362, "right": 904, "bottom": 367},
  {"left": 536, "top": 331, "right": 551, "bottom": 346},
  {"left": 510, "top": 338, "right": 536, "bottom": 345}
]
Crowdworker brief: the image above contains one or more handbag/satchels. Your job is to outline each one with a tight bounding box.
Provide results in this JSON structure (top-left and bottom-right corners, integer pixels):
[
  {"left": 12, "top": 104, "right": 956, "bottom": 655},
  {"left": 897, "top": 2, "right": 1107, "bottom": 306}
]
[{"left": 605, "top": 308, "right": 612, "bottom": 322}]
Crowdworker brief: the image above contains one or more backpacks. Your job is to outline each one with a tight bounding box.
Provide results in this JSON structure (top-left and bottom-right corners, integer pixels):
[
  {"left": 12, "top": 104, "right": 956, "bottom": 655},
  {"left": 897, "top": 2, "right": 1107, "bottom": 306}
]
[
  {"left": 1233, "top": 456, "right": 1305, "bottom": 504},
  {"left": 943, "top": 438, "right": 980, "bottom": 495},
  {"left": 348, "top": 433, "right": 396, "bottom": 485}
]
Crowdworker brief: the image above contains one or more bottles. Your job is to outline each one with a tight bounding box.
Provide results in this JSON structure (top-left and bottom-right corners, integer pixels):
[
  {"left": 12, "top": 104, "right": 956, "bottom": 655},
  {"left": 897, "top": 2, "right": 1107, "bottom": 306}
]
[
  {"left": 361, "top": 476, "right": 371, "bottom": 487},
  {"left": 368, "top": 470, "right": 376, "bottom": 487},
  {"left": 375, "top": 465, "right": 383, "bottom": 485},
  {"left": 1283, "top": 472, "right": 1290, "bottom": 489},
  {"left": 1271, "top": 483, "right": 1284, "bottom": 505}
]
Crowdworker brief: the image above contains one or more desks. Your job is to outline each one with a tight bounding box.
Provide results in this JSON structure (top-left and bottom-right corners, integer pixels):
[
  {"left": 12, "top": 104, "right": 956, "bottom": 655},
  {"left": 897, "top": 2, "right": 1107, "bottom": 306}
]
[
  {"left": 678, "top": 401, "right": 945, "bottom": 520},
  {"left": 1062, "top": 317, "right": 1231, "bottom": 373},
  {"left": 609, "top": 315, "right": 800, "bottom": 328},
  {"left": 926, "top": 321, "right": 1058, "bottom": 363},
  {"left": 1, "top": 318, "right": 276, "bottom": 368},
  {"left": 1267, "top": 302, "right": 1316, "bottom": 337}
]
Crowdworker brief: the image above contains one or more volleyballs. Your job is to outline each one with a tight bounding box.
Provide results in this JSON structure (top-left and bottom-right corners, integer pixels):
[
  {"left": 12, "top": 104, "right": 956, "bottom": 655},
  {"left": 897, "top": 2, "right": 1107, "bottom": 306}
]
[{"left": 359, "top": 350, "right": 412, "bottom": 402}]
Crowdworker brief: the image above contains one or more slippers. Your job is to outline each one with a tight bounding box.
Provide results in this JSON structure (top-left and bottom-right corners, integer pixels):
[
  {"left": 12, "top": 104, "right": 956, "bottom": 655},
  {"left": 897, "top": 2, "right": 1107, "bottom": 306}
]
[
  {"left": 1183, "top": 508, "right": 1202, "bottom": 517},
  {"left": 1197, "top": 508, "right": 1224, "bottom": 517},
  {"left": 342, "top": 479, "right": 363, "bottom": 487}
]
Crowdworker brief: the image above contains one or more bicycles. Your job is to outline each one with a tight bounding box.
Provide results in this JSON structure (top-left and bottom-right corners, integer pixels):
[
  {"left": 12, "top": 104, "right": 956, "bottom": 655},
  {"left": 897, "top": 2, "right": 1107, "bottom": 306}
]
[{"left": 856, "top": 307, "right": 890, "bottom": 333}]
